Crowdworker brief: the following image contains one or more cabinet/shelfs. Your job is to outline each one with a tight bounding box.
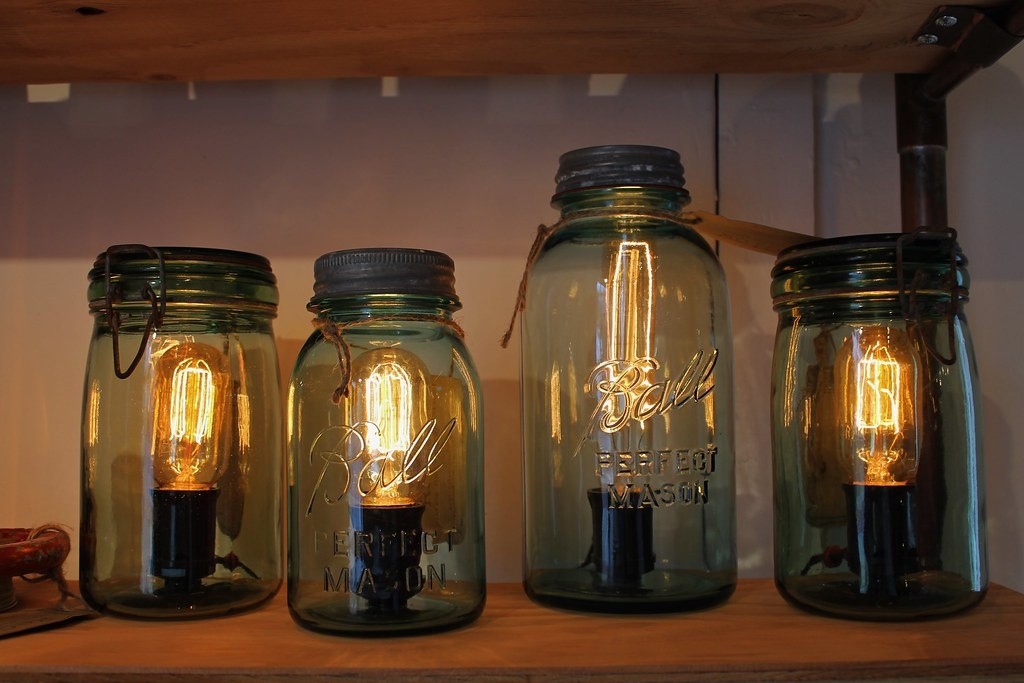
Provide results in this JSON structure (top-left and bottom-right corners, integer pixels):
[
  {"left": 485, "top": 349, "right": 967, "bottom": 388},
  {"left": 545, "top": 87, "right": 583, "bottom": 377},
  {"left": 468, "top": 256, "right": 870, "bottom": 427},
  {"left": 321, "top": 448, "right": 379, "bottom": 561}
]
[{"left": 0, "top": 0, "right": 1024, "bottom": 683}]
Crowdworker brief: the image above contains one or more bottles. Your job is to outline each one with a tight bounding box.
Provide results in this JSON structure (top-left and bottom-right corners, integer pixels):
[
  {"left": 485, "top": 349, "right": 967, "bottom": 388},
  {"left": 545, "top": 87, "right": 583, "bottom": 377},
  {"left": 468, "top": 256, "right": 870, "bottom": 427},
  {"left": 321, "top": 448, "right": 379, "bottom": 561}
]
[
  {"left": 285, "top": 248, "right": 487, "bottom": 638},
  {"left": 78, "top": 244, "right": 284, "bottom": 621},
  {"left": 769, "top": 229, "right": 991, "bottom": 622},
  {"left": 519, "top": 145, "right": 739, "bottom": 612}
]
